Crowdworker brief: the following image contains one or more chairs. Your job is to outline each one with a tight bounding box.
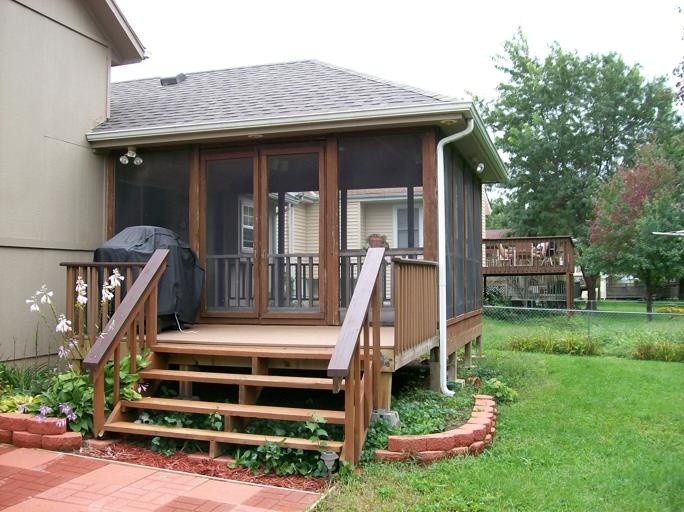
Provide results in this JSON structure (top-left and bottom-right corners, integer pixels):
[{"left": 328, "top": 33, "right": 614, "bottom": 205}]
[{"left": 496, "top": 241, "right": 549, "bottom": 267}]
